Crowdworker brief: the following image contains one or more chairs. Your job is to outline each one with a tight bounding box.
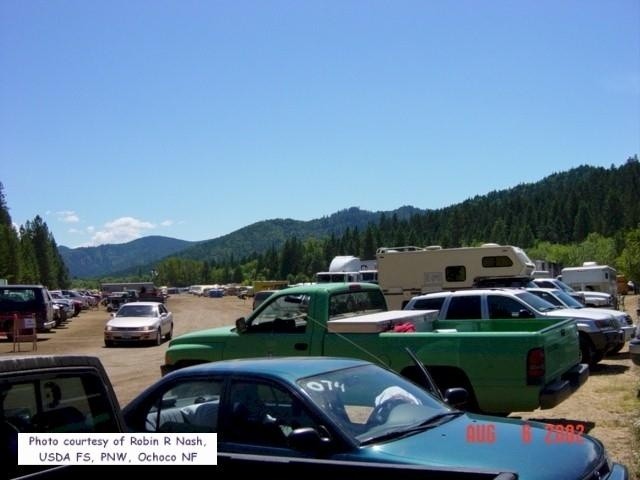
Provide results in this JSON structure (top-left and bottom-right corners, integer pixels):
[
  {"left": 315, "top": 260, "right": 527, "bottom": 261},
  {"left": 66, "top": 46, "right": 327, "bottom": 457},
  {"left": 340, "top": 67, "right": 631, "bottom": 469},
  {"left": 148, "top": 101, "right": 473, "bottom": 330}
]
[{"left": 292, "top": 388, "right": 349, "bottom": 423}]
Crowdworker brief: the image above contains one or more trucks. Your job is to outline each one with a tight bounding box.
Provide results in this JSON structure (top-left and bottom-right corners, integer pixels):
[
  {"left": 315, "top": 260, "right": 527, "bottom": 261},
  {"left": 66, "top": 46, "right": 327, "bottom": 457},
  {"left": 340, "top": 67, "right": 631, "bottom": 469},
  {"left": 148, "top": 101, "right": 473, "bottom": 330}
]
[
  {"left": 375, "top": 244, "right": 541, "bottom": 310},
  {"left": 554, "top": 261, "right": 618, "bottom": 306}
]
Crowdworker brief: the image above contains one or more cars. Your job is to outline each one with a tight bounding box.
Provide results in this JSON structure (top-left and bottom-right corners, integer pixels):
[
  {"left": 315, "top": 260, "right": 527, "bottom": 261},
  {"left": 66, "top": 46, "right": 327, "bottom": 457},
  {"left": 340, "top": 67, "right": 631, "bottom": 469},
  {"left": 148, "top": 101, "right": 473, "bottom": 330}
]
[
  {"left": 403, "top": 278, "right": 638, "bottom": 366},
  {"left": 189, "top": 283, "right": 253, "bottom": 297},
  {"left": 121, "top": 354, "right": 628, "bottom": 479},
  {"left": 0, "top": 284, "right": 102, "bottom": 340},
  {"left": 101, "top": 289, "right": 138, "bottom": 312},
  {"left": 104, "top": 301, "right": 174, "bottom": 346}
]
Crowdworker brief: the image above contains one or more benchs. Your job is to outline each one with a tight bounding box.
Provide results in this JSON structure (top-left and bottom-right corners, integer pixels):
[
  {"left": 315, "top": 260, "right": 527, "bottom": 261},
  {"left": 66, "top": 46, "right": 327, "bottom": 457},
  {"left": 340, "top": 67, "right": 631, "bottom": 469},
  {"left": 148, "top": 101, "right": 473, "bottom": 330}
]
[{"left": 145, "top": 393, "right": 276, "bottom": 437}]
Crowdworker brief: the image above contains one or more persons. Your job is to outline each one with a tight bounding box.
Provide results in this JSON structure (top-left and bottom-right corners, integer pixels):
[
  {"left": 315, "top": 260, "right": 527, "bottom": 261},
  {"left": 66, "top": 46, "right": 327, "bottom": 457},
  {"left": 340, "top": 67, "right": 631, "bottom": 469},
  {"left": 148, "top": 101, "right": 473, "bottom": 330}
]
[{"left": 36, "top": 381, "right": 70, "bottom": 411}]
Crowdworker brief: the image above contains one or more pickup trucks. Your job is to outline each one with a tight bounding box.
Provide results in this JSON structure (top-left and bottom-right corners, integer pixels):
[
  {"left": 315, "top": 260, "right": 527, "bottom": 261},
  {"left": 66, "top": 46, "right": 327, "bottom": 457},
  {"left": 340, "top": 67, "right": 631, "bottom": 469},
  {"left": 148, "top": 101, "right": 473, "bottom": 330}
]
[
  {"left": 160, "top": 281, "right": 590, "bottom": 417},
  {"left": 0, "top": 354, "right": 518, "bottom": 480}
]
